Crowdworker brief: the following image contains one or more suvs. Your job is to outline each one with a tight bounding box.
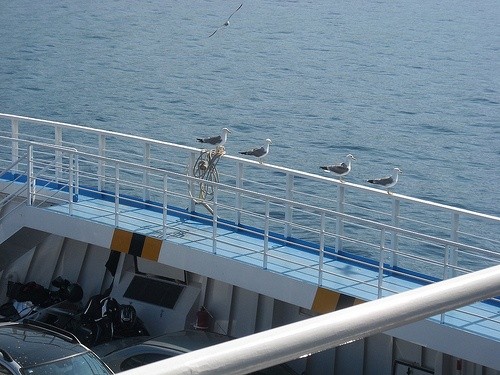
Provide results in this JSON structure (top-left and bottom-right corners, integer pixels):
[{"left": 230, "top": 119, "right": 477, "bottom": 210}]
[{"left": 1, "top": 319, "right": 114, "bottom": 374}]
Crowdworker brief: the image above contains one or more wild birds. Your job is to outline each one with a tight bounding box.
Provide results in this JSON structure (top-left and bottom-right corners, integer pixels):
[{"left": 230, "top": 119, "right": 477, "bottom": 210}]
[
  {"left": 320, "top": 153, "right": 356, "bottom": 183},
  {"left": 207, "top": 3, "right": 243, "bottom": 38},
  {"left": 239, "top": 138, "right": 273, "bottom": 164},
  {"left": 366, "top": 168, "right": 403, "bottom": 195},
  {"left": 195, "top": 128, "right": 231, "bottom": 146}
]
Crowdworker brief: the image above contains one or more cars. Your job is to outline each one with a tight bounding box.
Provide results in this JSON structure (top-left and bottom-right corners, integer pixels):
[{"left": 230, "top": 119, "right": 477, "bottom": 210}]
[
  {"left": 6, "top": 277, "right": 148, "bottom": 337},
  {"left": 91, "top": 329, "right": 297, "bottom": 375}
]
